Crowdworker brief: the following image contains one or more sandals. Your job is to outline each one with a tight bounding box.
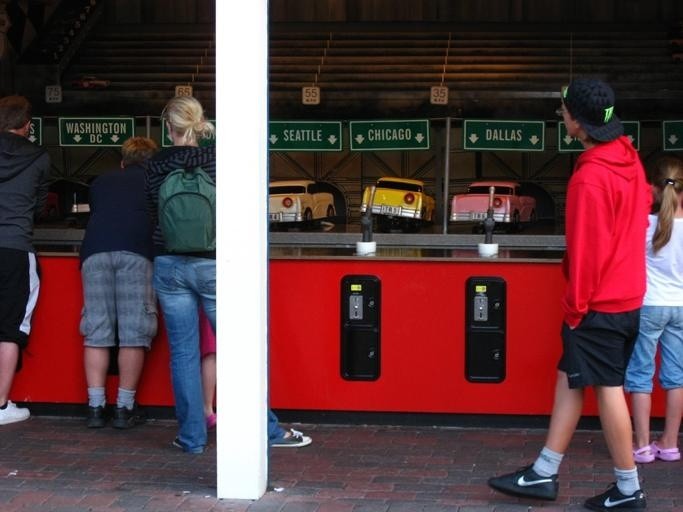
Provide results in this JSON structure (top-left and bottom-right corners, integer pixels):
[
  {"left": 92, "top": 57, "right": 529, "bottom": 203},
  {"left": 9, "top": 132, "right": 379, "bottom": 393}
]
[
  {"left": 649, "top": 439, "right": 682, "bottom": 462},
  {"left": 631, "top": 443, "right": 656, "bottom": 464}
]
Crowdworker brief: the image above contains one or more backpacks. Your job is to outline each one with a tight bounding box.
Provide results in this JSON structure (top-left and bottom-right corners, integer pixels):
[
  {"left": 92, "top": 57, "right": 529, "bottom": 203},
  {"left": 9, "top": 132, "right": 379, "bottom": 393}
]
[{"left": 156, "top": 164, "right": 216, "bottom": 254}]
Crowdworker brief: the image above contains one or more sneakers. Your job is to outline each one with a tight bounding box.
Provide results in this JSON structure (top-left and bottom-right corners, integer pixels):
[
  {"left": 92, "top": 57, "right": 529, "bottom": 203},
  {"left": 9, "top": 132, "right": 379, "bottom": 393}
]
[
  {"left": 172, "top": 436, "right": 204, "bottom": 454},
  {"left": 86, "top": 402, "right": 111, "bottom": 428},
  {"left": 113, "top": 400, "right": 148, "bottom": 428},
  {"left": 0, "top": 399, "right": 30, "bottom": 426},
  {"left": 270, "top": 427, "right": 313, "bottom": 449},
  {"left": 486, "top": 462, "right": 560, "bottom": 502},
  {"left": 583, "top": 479, "right": 647, "bottom": 512}
]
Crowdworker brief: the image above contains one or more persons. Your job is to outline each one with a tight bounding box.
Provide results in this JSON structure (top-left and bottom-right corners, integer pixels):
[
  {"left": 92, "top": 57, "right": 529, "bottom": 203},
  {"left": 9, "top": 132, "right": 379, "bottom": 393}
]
[
  {"left": 148, "top": 94, "right": 314, "bottom": 456},
  {"left": 195, "top": 302, "right": 216, "bottom": 427},
  {"left": 72, "top": 136, "right": 160, "bottom": 432},
  {"left": 0, "top": 95, "right": 83, "bottom": 429},
  {"left": 486, "top": 77, "right": 652, "bottom": 511},
  {"left": 621, "top": 166, "right": 683, "bottom": 466}
]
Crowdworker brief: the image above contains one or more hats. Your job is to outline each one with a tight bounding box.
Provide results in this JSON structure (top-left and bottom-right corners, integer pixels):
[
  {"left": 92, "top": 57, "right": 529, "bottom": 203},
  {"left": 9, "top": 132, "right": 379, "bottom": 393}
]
[{"left": 562, "top": 77, "right": 624, "bottom": 143}]
